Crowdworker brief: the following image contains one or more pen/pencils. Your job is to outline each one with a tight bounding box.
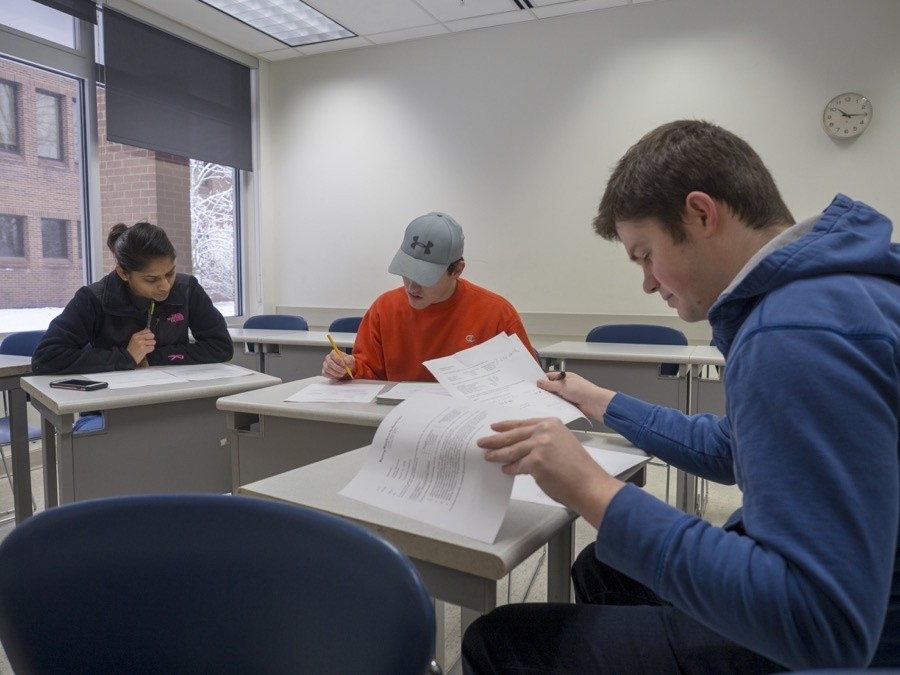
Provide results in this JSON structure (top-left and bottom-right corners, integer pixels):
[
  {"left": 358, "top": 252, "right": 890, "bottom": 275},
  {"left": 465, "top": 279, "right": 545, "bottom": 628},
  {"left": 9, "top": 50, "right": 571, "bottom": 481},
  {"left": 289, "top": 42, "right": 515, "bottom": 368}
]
[
  {"left": 554, "top": 371, "right": 566, "bottom": 382},
  {"left": 327, "top": 334, "right": 353, "bottom": 379},
  {"left": 147, "top": 300, "right": 155, "bottom": 329}
]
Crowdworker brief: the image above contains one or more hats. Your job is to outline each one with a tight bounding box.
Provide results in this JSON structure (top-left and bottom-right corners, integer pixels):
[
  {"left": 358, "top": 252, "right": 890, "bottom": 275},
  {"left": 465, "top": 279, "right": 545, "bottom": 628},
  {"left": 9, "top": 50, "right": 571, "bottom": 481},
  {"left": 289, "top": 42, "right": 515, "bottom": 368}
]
[{"left": 388, "top": 210, "right": 466, "bottom": 288}]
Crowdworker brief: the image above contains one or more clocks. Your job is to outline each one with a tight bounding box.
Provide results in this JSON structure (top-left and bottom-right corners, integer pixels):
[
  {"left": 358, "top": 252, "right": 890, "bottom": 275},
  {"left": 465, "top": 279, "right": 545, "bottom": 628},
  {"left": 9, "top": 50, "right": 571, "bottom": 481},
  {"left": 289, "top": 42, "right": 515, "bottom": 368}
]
[{"left": 823, "top": 92, "right": 873, "bottom": 141}]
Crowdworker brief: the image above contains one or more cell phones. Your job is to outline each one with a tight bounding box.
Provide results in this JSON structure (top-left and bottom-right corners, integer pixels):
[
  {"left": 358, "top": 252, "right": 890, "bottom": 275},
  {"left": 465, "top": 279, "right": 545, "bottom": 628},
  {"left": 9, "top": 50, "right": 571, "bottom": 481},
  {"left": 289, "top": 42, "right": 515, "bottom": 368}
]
[{"left": 49, "top": 378, "right": 108, "bottom": 391}]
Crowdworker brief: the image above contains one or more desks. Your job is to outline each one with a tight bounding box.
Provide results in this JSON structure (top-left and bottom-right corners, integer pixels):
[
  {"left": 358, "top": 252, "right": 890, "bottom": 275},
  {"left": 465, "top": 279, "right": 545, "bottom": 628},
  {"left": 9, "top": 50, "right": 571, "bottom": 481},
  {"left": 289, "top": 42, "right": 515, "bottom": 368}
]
[{"left": 0, "top": 340, "right": 726, "bottom": 675}]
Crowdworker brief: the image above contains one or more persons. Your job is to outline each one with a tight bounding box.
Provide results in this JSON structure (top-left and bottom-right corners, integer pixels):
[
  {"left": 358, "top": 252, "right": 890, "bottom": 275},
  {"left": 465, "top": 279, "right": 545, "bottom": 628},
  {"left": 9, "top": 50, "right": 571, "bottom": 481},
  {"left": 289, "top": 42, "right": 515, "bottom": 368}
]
[
  {"left": 30, "top": 219, "right": 234, "bottom": 376},
  {"left": 322, "top": 212, "right": 541, "bottom": 383},
  {"left": 463, "top": 120, "right": 900, "bottom": 675}
]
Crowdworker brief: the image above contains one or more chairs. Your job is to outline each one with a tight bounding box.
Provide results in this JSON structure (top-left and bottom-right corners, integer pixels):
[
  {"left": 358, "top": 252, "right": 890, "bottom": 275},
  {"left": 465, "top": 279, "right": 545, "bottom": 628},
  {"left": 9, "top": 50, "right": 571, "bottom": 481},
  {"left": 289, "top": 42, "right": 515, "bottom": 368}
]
[
  {"left": 242, "top": 314, "right": 309, "bottom": 354},
  {"left": 582, "top": 325, "right": 688, "bottom": 505},
  {"left": 0, "top": 330, "right": 50, "bottom": 414},
  {"left": 328, "top": 316, "right": 365, "bottom": 332},
  {"left": 0, "top": 387, "right": 42, "bottom": 525},
  {"left": 0, "top": 491, "right": 437, "bottom": 675}
]
